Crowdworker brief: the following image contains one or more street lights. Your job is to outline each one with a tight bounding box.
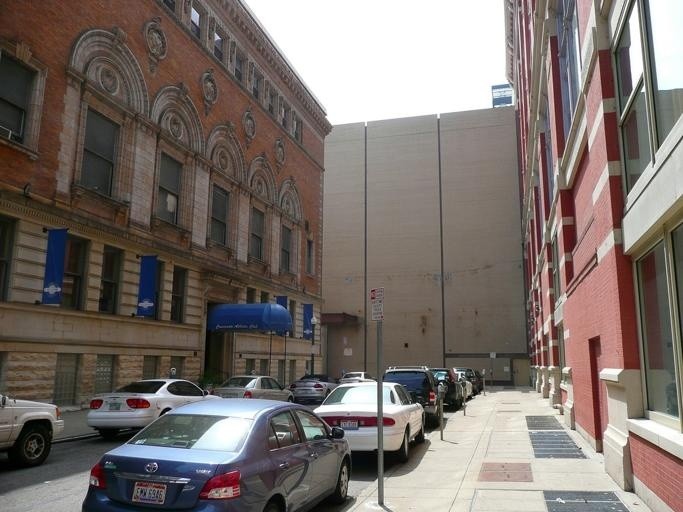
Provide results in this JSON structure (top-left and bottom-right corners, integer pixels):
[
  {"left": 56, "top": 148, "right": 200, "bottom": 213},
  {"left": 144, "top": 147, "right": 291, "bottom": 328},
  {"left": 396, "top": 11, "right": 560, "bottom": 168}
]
[{"left": 309, "top": 315, "right": 318, "bottom": 378}]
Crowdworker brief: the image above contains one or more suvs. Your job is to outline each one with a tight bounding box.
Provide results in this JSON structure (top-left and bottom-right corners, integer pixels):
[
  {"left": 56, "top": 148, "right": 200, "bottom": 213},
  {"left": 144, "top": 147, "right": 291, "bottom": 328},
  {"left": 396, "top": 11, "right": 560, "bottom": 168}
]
[{"left": 0, "top": 391, "right": 68, "bottom": 473}]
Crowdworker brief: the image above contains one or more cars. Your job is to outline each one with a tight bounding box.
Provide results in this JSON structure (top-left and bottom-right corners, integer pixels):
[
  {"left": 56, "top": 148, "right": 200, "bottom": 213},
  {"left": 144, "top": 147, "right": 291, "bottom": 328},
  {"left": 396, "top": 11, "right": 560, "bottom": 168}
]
[
  {"left": 208, "top": 363, "right": 483, "bottom": 469},
  {"left": 80, "top": 375, "right": 222, "bottom": 440},
  {"left": 75, "top": 396, "right": 352, "bottom": 512}
]
